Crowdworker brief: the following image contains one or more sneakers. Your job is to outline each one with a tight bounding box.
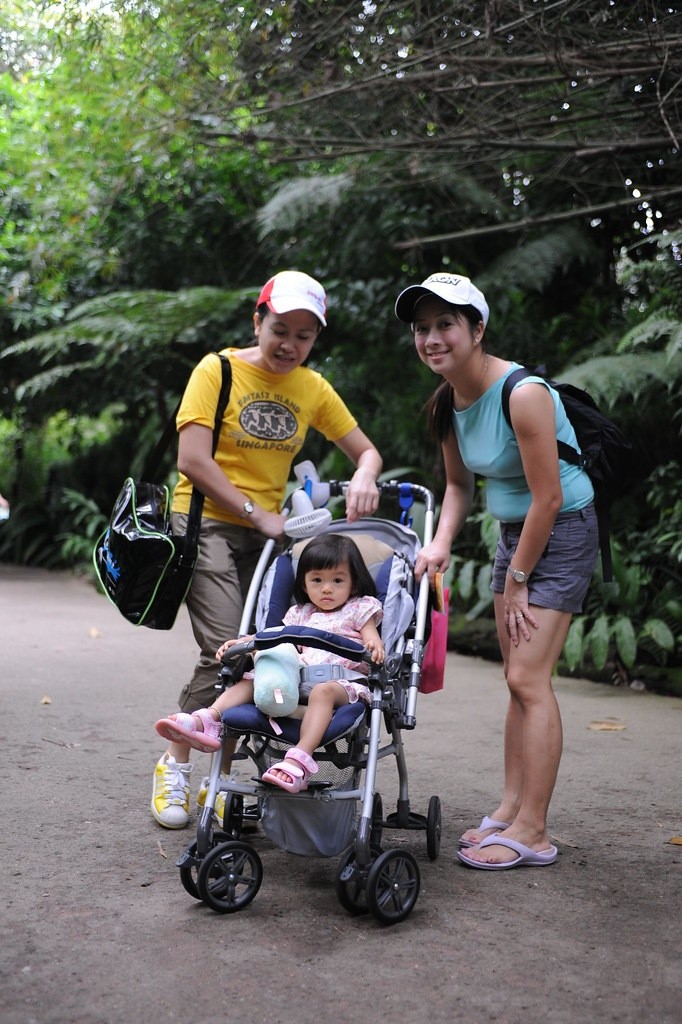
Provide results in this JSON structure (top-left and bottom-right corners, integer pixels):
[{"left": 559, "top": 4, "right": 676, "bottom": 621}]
[
  {"left": 197, "top": 772, "right": 246, "bottom": 829},
  {"left": 151, "top": 752, "right": 193, "bottom": 829}
]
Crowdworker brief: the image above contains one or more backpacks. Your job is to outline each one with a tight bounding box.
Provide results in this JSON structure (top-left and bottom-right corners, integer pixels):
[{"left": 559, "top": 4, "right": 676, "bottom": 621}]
[{"left": 501, "top": 358, "right": 641, "bottom": 501}]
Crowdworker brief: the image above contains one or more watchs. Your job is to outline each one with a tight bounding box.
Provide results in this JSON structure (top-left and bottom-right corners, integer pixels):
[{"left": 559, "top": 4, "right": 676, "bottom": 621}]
[
  {"left": 238, "top": 500, "right": 254, "bottom": 520},
  {"left": 507, "top": 566, "right": 529, "bottom": 584}
]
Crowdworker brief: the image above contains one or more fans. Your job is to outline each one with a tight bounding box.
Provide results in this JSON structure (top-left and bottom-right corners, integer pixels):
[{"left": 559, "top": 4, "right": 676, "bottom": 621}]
[{"left": 284, "top": 459, "right": 333, "bottom": 539}]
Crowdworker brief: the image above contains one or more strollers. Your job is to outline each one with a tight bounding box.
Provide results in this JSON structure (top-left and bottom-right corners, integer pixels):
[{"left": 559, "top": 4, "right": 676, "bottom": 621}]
[{"left": 175, "top": 478, "right": 444, "bottom": 926}]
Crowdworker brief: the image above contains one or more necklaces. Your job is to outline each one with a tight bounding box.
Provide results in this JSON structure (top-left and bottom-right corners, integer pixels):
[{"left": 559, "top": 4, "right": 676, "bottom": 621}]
[{"left": 453, "top": 355, "right": 488, "bottom": 395}]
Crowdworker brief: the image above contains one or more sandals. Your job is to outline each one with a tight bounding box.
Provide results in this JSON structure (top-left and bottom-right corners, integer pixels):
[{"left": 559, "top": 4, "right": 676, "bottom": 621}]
[
  {"left": 262, "top": 747, "right": 319, "bottom": 794},
  {"left": 154, "top": 707, "right": 224, "bottom": 753}
]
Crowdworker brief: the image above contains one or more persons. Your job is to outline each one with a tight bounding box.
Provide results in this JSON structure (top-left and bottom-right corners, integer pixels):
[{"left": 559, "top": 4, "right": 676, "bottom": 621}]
[
  {"left": 396, "top": 273, "right": 600, "bottom": 870},
  {"left": 156, "top": 533, "right": 385, "bottom": 794},
  {"left": 150, "top": 270, "right": 382, "bottom": 829}
]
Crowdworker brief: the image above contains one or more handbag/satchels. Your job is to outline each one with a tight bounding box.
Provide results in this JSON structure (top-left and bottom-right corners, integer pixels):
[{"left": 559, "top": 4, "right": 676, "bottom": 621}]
[
  {"left": 418, "top": 586, "right": 450, "bottom": 694},
  {"left": 91, "top": 476, "right": 200, "bottom": 630}
]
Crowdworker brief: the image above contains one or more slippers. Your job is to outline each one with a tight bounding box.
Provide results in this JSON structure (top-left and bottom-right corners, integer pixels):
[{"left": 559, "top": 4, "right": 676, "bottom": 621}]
[
  {"left": 458, "top": 815, "right": 512, "bottom": 848},
  {"left": 456, "top": 833, "right": 559, "bottom": 870}
]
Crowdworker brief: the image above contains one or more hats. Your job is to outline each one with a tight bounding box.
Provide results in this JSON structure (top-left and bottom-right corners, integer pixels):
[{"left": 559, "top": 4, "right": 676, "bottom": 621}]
[
  {"left": 394, "top": 272, "right": 490, "bottom": 333},
  {"left": 255, "top": 271, "right": 326, "bottom": 327}
]
